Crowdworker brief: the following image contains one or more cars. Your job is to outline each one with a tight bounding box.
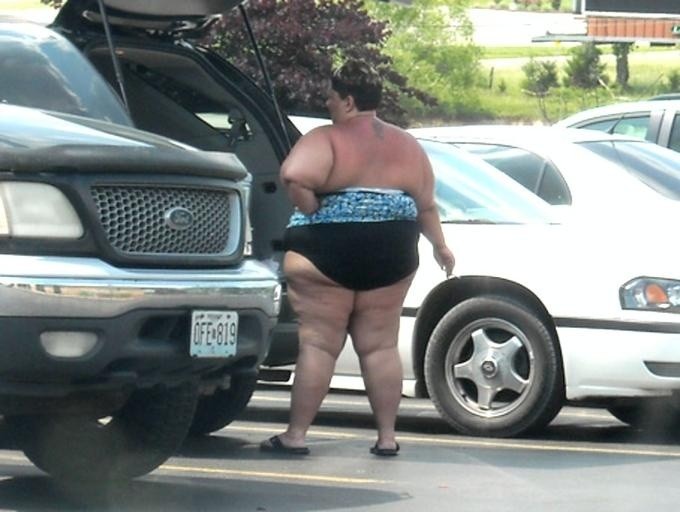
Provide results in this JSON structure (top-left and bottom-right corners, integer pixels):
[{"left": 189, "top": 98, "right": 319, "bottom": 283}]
[
  {"left": 401, "top": 124, "right": 680, "bottom": 216},
  {"left": 188, "top": 113, "right": 680, "bottom": 438}
]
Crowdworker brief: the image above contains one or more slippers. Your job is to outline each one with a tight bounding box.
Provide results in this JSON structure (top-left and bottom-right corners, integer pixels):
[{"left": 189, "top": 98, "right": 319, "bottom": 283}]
[
  {"left": 370, "top": 441, "right": 399, "bottom": 455},
  {"left": 260, "top": 435, "right": 309, "bottom": 453}
]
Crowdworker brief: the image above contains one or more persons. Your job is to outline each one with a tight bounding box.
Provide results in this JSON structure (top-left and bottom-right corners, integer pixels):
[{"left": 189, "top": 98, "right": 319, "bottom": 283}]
[{"left": 260, "top": 58, "right": 456, "bottom": 457}]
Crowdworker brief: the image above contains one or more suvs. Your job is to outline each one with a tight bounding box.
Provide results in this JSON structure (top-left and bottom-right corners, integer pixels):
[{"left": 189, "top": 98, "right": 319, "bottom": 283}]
[
  {"left": 553, "top": 94, "right": 680, "bottom": 154},
  {"left": 0, "top": 20, "right": 284, "bottom": 480},
  {"left": 38, "top": 2, "right": 330, "bottom": 442}
]
